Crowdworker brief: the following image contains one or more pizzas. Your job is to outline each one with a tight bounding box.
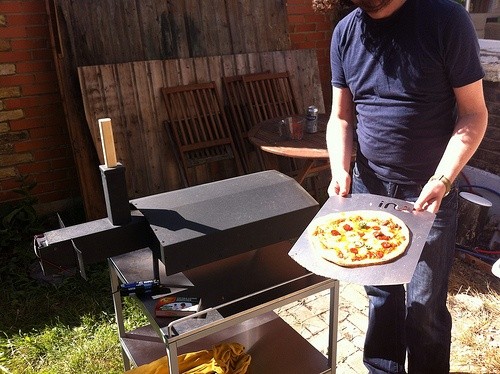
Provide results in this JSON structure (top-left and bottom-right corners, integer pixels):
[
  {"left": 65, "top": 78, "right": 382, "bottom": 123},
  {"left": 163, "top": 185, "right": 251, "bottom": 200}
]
[{"left": 308, "top": 210, "right": 410, "bottom": 267}]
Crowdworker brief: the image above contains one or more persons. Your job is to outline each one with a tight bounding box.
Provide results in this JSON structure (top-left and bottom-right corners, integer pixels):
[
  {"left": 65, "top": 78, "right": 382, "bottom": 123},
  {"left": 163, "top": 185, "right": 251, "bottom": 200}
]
[{"left": 309, "top": 0, "right": 488, "bottom": 374}]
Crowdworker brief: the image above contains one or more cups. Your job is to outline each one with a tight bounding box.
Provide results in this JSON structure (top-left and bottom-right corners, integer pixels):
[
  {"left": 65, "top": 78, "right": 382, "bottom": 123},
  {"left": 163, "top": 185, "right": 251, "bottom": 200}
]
[{"left": 284, "top": 116, "right": 306, "bottom": 141}]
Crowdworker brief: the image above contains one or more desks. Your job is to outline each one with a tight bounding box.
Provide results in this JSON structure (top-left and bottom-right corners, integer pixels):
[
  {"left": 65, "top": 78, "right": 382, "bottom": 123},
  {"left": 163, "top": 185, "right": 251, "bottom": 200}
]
[{"left": 247, "top": 113, "right": 331, "bottom": 184}]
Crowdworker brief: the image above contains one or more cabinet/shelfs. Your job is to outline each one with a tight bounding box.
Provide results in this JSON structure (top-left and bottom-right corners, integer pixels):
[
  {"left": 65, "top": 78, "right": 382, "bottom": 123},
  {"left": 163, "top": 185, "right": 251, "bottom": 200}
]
[{"left": 108, "top": 247, "right": 339, "bottom": 374}]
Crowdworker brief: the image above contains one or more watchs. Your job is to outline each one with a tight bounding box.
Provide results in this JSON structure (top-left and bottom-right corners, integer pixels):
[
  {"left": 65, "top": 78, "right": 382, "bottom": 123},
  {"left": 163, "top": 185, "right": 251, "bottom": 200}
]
[{"left": 427, "top": 175, "right": 452, "bottom": 198}]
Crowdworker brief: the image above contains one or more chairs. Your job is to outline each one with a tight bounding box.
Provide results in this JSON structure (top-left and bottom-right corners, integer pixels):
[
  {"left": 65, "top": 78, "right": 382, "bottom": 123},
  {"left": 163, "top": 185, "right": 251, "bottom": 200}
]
[
  {"left": 242, "top": 70, "right": 319, "bottom": 204},
  {"left": 161, "top": 81, "right": 245, "bottom": 188},
  {"left": 224, "top": 73, "right": 309, "bottom": 192}
]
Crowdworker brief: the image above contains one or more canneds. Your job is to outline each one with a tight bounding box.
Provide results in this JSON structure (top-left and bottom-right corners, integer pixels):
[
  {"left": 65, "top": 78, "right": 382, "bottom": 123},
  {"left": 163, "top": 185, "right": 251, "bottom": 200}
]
[{"left": 305, "top": 105, "right": 319, "bottom": 133}]
